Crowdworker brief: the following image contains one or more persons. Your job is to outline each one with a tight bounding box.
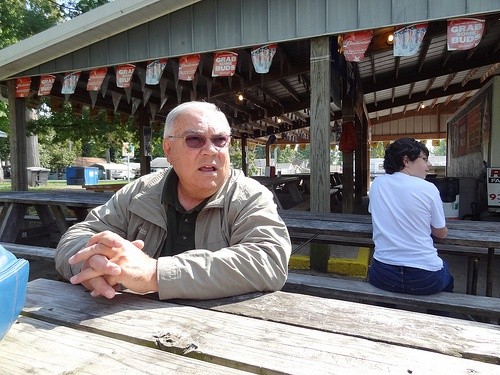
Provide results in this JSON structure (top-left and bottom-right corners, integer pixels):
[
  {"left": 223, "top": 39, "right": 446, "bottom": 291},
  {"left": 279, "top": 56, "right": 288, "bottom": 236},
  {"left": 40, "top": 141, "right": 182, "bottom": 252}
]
[
  {"left": 54, "top": 101, "right": 292, "bottom": 299},
  {"left": 368, "top": 137, "right": 454, "bottom": 316}
]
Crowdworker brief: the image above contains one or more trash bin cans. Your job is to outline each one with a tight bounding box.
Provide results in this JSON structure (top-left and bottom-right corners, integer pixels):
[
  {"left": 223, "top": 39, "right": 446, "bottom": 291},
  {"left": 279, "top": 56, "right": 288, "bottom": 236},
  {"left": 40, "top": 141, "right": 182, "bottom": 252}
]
[
  {"left": 26, "top": 167, "right": 52, "bottom": 188},
  {"left": 66, "top": 165, "right": 100, "bottom": 186}
]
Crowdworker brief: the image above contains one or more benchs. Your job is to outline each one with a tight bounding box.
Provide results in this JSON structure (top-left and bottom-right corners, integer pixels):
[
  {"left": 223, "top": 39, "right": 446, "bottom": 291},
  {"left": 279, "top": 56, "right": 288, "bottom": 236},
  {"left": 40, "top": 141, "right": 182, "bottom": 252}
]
[
  {"left": 287, "top": 189, "right": 340, "bottom": 211},
  {"left": 0, "top": 238, "right": 500, "bottom": 324}
]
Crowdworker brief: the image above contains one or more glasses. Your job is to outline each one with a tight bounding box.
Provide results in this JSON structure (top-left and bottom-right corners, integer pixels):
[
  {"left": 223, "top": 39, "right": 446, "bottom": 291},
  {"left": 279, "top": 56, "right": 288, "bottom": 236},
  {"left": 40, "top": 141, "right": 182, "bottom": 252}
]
[
  {"left": 419, "top": 155, "right": 429, "bottom": 163},
  {"left": 168, "top": 133, "right": 229, "bottom": 150}
]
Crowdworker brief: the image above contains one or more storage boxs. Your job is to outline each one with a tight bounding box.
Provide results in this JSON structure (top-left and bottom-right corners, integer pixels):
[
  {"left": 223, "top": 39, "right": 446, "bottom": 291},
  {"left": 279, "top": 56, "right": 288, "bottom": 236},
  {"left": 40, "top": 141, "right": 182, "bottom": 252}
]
[{"left": 66, "top": 167, "right": 99, "bottom": 185}]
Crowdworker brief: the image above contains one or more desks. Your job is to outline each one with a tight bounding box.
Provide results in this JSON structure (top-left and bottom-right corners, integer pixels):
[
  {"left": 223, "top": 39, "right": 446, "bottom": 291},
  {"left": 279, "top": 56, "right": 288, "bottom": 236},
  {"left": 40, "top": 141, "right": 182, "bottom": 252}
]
[
  {"left": 0, "top": 279, "right": 500, "bottom": 375},
  {"left": 0, "top": 186, "right": 500, "bottom": 296},
  {"left": 251, "top": 176, "right": 304, "bottom": 211}
]
